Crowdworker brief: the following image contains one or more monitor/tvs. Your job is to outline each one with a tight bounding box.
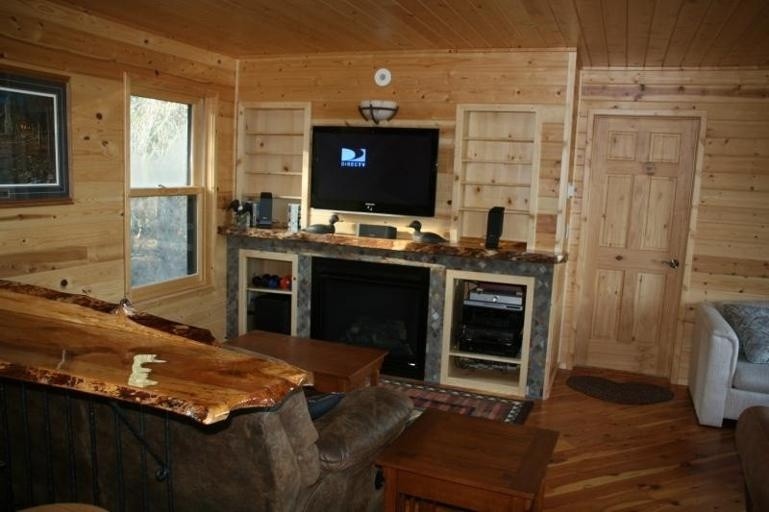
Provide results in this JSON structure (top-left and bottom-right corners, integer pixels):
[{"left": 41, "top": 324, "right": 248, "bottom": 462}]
[{"left": 310, "top": 124, "right": 440, "bottom": 218}]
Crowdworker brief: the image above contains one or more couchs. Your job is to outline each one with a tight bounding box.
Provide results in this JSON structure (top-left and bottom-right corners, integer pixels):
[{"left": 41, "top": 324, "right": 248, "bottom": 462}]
[
  {"left": 688, "top": 300, "right": 769, "bottom": 428},
  {"left": 733, "top": 405, "right": 768, "bottom": 512},
  {"left": 0, "top": 280, "right": 414, "bottom": 511}
]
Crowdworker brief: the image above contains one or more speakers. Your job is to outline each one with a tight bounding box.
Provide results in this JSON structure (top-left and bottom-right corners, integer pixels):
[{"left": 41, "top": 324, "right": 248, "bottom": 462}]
[
  {"left": 485, "top": 205, "right": 506, "bottom": 250},
  {"left": 257, "top": 192, "right": 273, "bottom": 228}
]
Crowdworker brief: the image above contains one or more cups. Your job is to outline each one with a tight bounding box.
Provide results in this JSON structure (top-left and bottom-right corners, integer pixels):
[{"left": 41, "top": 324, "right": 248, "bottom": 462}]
[{"left": 287, "top": 202, "right": 301, "bottom": 232}]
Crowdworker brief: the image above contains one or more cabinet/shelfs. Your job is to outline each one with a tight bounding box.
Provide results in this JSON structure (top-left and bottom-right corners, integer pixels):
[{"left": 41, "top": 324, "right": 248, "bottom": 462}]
[
  {"left": 235, "top": 101, "right": 311, "bottom": 229},
  {"left": 441, "top": 269, "right": 535, "bottom": 399},
  {"left": 374, "top": 407, "right": 559, "bottom": 512},
  {"left": 238, "top": 248, "right": 297, "bottom": 337},
  {"left": 451, "top": 103, "right": 544, "bottom": 252}
]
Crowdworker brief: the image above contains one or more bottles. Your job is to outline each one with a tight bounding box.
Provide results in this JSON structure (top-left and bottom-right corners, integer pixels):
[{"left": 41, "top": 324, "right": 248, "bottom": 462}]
[{"left": 258, "top": 191, "right": 274, "bottom": 229}]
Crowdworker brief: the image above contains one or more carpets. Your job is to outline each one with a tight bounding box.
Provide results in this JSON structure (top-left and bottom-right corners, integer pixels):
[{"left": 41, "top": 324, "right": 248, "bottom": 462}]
[
  {"left": 566, "top": 375, "right": 673, "bottom": 405},
  {"left": 381, "top": 377, "right": 532, "bottom": 426}
]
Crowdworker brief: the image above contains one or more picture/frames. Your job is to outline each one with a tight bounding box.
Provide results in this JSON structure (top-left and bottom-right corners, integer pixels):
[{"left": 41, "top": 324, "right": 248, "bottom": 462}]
[{"left": 0, "top": 65, "right": 74, "bottom": 208}]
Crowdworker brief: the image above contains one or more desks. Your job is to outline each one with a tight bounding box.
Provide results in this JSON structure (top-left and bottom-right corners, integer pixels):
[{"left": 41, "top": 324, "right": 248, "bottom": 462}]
[{"left": 221, "top": 330, "right": 388, "bottom": 393}]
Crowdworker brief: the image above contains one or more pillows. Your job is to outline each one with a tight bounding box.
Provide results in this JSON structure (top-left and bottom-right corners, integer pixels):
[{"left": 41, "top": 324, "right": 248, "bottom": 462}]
[{"left": 723, "top": 303, "right": 769, "bottom": 365}]
